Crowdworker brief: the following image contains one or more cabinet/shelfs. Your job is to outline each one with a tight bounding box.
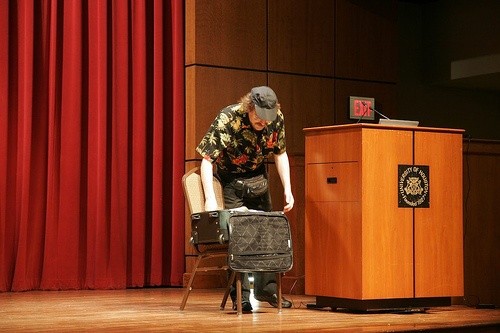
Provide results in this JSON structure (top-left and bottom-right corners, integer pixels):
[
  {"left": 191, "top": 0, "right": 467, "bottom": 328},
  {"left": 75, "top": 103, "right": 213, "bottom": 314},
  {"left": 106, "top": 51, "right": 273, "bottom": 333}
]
[{"left": 303, "top": 123, "right": 466, "bottom": 312}]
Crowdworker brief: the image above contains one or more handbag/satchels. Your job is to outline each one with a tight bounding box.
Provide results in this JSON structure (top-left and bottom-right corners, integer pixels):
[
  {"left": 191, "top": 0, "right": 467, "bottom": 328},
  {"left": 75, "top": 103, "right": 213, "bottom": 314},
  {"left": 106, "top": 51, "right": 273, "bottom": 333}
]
[{"left": 234, "top": 174, "right": 269, "bottom": 200}]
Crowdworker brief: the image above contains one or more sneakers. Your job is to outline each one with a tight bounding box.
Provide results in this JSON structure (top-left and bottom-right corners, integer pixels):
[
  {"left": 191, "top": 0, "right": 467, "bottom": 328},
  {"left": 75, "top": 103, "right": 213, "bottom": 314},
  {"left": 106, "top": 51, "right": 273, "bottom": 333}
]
[
  {"left": 256, "top": 292, "right": 292, "bottom": 308},
  {"left": 233, "top": 295, "right": 253, "bottom": 311}
]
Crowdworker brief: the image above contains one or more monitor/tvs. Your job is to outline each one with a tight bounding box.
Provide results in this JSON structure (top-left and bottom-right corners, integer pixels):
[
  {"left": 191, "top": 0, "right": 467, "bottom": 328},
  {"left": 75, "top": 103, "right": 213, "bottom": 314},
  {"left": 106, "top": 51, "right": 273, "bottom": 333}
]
[{"left": 379, "top": 119, "right": 419, "bottom": 127}]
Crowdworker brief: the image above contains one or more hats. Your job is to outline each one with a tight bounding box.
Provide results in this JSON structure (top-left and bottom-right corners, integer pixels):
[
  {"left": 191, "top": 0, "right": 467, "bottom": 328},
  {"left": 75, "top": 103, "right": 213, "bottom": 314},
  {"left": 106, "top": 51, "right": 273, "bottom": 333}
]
[{"left": 250, "top": 86, "right": 278, "bottom": 120}]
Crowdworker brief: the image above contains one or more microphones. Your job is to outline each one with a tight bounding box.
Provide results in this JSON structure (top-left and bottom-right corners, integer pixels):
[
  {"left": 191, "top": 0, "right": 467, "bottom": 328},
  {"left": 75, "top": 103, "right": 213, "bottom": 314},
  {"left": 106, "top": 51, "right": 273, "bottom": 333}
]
[
  {"left": 369, "top": 106, "right": 389, "bottom": 120},
  {"left": 357, "top": 101, "right": 368, "bottom": 123}
]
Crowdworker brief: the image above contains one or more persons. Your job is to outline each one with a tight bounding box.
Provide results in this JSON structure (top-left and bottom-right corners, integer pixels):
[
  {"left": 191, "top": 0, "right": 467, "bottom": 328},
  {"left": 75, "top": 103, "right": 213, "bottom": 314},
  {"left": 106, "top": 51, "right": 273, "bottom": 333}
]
[{"left": 195, "top": 86, "right": 295, "bottom": 311}]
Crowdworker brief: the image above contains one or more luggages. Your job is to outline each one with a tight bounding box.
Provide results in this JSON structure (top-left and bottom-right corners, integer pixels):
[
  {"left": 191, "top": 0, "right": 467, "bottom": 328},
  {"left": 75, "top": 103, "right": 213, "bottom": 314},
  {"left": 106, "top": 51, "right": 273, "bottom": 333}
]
[{"left": 188, "top": 209, "right": 293, "bottom": 273}]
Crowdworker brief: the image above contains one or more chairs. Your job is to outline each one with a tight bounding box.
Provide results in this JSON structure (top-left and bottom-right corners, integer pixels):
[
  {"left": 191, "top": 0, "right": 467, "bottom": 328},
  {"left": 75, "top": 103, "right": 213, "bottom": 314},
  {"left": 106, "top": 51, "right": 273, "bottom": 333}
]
[{"left": 179, "top": 166, "right": 286, "bottom": 316}]
